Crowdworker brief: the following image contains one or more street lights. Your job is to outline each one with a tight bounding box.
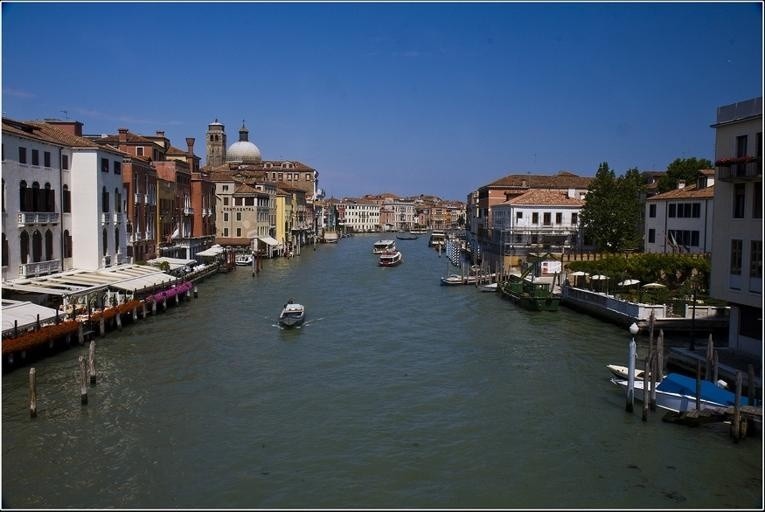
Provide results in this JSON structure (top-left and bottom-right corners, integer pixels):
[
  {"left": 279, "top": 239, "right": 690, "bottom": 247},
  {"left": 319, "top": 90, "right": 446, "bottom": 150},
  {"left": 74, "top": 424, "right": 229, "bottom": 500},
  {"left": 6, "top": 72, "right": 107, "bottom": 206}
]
[{"left": 690, "top": 283, "right": 702, "bottom": 352}]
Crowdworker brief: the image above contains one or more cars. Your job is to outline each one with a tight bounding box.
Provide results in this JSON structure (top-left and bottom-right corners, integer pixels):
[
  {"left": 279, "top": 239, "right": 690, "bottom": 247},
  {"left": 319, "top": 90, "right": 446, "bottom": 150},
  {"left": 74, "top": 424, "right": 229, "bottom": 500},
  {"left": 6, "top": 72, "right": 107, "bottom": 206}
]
[{"left": 367, "top": 228, "right": 375, "bottom": 233}]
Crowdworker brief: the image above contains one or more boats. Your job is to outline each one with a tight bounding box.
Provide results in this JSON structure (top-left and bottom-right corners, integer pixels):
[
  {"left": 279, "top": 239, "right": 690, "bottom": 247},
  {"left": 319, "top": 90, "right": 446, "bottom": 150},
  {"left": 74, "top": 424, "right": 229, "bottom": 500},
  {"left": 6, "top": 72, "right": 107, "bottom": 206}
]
[
  {"left": 379, "top": 250, "right": 403, "bottom": 267},
  {"left": 279, "top": 298, "right": 306, "bottom": 328},
  {"left": 607, "top": 379, "right": 758, "bottom": 414},
  {"left": 373, "top": 239, "right": 396, "bottom": 254},
  {"left": 441, "top": 271, "right": 497, "bottom": 285},
  {"left": 235, "top": 253, "right": 255, "bottom": 267},
  {"left": 428, "top": 230, "right": 446, "bottom": 249},
  {"left": 497, "top": 252, "right": 563, "bottom": 311},
  {"left": 478, "top": 281, "right": 523, "bottom": 292},
  {"left": 410, "top": 229, "right": 427, "bottom": 235},
  {"left": 607, "top": 365, "right": 760, "bottom": 408},
  {"left": 396, "top": 234, "right": 420, "bottom": 241}
]
